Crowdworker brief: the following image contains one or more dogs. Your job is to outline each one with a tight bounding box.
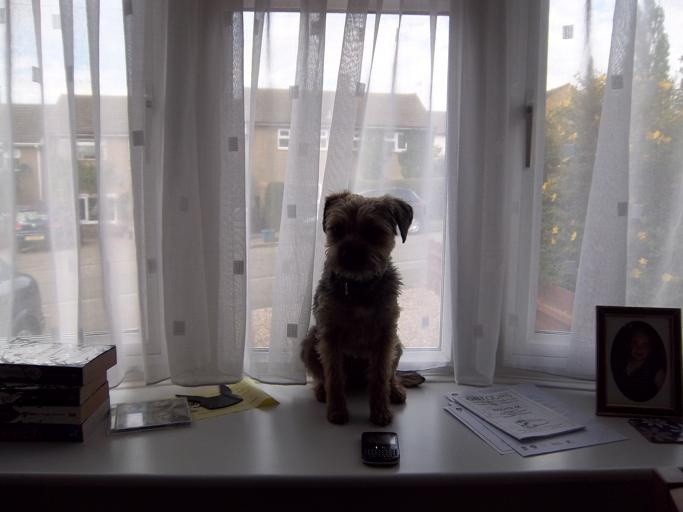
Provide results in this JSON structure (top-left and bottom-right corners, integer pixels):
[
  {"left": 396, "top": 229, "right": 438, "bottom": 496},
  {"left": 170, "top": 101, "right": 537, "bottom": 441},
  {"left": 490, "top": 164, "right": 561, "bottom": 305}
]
[{"left": 300, "top": 189, "right": 415, "bottom": 427}]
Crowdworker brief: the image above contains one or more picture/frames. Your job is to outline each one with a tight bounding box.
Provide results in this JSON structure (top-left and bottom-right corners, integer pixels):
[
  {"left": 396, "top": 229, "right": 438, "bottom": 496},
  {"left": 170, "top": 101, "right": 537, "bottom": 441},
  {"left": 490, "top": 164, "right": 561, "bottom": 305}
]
[{"left": 594, "top": 304, "right": 683, "bottom": 422}]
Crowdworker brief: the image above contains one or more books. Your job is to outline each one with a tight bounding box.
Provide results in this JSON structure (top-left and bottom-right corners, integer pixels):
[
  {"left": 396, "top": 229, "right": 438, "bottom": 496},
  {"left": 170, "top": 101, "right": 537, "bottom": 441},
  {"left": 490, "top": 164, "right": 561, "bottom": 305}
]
[
  {"left": 452, "top": 388, "right": 586, "bottom": 443},
  {"left": 106, "top": 394, "right": 191, "bottom": 435},
  {"left": 0, "top": 334, "right": 115, "bottom": 443}
]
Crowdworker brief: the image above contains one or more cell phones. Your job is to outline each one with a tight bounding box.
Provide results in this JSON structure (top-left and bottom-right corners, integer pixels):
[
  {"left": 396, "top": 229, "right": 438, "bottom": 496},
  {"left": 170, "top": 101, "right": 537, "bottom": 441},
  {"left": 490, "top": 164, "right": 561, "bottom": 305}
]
[{"left": 360, "top": 432, "right": 400, "bottom": 467}]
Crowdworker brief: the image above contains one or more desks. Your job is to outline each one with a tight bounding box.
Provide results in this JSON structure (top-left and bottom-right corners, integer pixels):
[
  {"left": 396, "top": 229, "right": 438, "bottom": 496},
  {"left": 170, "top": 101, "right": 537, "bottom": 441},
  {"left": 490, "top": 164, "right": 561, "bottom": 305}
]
[{"left": 1, "top": 376, "right": 682, "bottom": 512}]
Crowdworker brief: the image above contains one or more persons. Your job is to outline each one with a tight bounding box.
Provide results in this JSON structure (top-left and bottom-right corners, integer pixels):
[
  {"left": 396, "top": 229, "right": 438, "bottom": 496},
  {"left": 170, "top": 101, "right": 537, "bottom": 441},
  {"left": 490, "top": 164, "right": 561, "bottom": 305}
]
[{"left": 611, "top": 327, "right": 665, "bottom": 402}]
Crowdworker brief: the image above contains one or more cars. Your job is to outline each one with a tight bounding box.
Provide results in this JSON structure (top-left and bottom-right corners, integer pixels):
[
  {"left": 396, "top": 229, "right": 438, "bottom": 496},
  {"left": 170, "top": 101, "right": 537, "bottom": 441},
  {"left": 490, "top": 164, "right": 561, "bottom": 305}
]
[
  {"left": 301, "top": 187, "right": 428, "bottom": 235},
  {"left": 13, "top": 205, "right": 83, "bottom": 249},
  {"left": 0, "top": 260, "right": 45, "bottom": 337}
]
